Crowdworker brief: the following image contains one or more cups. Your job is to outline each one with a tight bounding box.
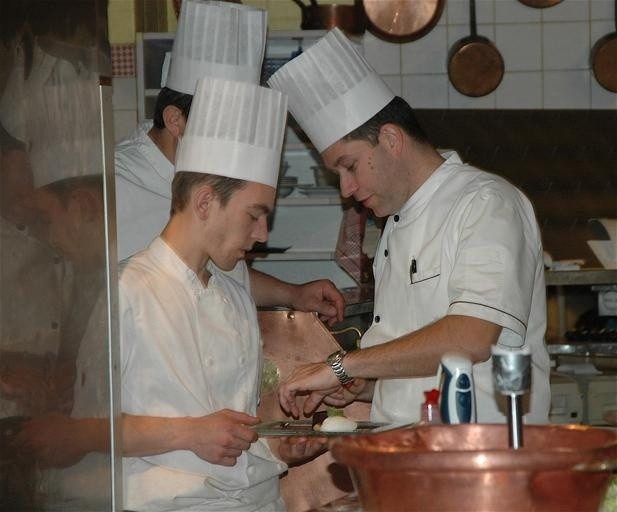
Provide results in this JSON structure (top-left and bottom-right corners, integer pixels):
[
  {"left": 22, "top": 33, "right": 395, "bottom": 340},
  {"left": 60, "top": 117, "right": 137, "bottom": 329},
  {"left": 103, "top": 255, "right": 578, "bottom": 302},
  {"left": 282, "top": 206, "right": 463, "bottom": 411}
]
[{"left": 309, "top": 165, "right": 336, "bottom": 185}]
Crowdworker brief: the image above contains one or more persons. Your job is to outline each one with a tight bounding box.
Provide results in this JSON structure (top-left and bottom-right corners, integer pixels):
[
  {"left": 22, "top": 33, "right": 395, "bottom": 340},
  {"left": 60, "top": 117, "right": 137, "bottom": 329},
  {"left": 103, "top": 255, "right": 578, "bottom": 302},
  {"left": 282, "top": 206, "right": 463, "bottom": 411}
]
[
  {"left": 114, "top": 88, "right": 351, "bottom": 333},
  {"left": 11, "top": 174, "right": 113, "bottom": 511},
  {"left": 118, "top": 169, "right": 339, "bottom": 512},
  {"left": 0, "top": 123, "right": 71, "bottom": 510},
  {"left": 274, "top": 92, "right": 553, "bottom": 427}
]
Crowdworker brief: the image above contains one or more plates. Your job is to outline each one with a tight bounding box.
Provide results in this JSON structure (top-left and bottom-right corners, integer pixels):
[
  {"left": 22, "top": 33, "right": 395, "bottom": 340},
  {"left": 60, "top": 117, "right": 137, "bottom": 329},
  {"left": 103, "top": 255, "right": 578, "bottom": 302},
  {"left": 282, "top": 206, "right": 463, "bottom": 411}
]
[
  {"left": 275, "top": 157, "right": 298, "bottom": 199},
  {"left": 297, "top": 182, "right": 340, "bottom": 197}
]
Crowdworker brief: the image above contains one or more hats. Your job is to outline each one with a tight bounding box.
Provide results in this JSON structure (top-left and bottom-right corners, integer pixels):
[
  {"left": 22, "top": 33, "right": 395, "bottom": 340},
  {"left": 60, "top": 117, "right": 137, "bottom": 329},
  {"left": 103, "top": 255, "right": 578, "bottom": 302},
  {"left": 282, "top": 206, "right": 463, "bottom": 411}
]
[
  {"left": 173, "top": 77, "right": 287, "bottom": 190},
  {"left": 165, "top": 1, "right": 268, "bottom": 96},
  {"left": 266, "top": 25, "right": 397, "bottom": 156}
]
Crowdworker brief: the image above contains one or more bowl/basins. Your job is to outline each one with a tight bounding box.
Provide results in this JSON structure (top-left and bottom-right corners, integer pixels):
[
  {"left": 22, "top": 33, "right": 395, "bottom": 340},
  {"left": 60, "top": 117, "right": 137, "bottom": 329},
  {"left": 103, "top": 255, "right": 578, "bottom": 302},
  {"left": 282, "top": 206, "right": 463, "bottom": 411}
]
[
  {"left": 589, "top": 351, "right": 617, "bottom": 374},
  {"left": 327, "top": 421, "right": 617, "bottom": 512}
]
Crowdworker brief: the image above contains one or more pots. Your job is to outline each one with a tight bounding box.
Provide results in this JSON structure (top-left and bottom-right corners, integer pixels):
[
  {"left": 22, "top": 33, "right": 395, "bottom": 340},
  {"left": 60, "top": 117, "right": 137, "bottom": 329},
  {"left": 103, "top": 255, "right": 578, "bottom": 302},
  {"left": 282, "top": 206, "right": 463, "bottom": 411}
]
[
  {"left": 295, "top": 2, "right": 444, "bottom": 45},
  {"left": 589, "top": 3, "right": 616, "bottom": 92},
  {"left": 446, "top": 1, "right": 504, "bottom": 97}
]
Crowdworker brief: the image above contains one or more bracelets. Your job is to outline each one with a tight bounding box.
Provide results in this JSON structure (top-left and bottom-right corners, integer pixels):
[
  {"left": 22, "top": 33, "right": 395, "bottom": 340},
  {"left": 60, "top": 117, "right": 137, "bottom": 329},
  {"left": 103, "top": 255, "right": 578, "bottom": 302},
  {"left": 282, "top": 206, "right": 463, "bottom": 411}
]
[{"left": 324, "top": 347, "right": 356, "bottom": 391}]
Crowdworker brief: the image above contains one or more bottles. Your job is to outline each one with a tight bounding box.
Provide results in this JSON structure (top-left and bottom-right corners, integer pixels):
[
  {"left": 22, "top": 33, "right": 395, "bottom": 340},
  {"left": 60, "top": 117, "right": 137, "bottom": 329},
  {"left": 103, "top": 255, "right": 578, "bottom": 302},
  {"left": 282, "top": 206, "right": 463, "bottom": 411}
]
[{"left": 416, "top": 388, "right": 445, "bottom": 426}]
